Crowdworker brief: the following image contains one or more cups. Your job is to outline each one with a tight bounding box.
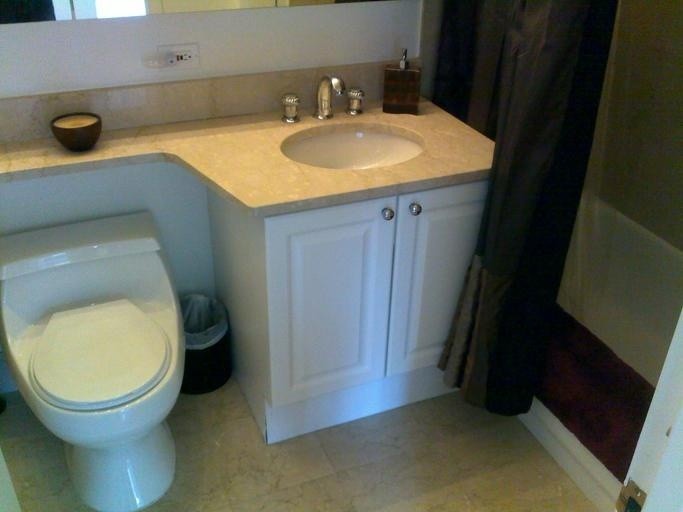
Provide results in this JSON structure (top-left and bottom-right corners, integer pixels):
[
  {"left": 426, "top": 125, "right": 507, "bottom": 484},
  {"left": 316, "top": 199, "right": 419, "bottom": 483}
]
[{"left": 49, "top": 111, "right": 102, "bottom": 153}]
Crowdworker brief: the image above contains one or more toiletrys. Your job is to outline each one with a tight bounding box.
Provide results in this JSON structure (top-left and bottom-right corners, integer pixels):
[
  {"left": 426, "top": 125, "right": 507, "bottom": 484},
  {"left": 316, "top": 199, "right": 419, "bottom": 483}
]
[{"left": 382, "top": 49, "right": 420, "bottom": 115}]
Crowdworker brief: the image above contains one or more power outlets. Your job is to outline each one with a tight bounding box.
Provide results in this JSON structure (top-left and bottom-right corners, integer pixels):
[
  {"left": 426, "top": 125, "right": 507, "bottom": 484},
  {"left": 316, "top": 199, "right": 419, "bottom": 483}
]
[{"left": 155, "top": 42, "right": 202, "bottom": 70}]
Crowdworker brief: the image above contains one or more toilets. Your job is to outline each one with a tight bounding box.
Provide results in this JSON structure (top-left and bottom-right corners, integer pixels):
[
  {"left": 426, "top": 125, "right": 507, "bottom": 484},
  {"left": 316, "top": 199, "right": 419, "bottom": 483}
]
[{"left": 0, "top": 211, "right": 186, "bottom": 512}]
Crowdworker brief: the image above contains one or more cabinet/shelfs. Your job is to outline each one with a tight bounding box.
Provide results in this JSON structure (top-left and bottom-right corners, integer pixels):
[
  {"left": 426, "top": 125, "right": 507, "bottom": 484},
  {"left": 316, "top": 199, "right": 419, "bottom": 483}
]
[{"left": 209, "top": 180, "right": 492, "bottom": 445}]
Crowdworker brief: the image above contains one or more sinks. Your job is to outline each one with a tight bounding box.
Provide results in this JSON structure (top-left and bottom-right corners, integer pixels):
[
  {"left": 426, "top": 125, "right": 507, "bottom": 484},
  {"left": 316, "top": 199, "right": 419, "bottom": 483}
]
[{"left": 279, "top": 123, "right": 426, "bottom": 170}]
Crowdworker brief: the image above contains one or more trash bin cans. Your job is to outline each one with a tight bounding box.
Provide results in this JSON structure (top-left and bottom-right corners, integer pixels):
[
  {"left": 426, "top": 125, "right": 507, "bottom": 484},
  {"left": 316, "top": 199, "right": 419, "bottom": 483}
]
[{"left": 177, "top": 293, "right": 232, "bottom": 394}]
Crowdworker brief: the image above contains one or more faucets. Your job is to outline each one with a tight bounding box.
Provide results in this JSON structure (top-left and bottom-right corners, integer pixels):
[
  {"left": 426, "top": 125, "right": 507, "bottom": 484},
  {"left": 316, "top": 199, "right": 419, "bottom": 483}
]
[{"left": 314, "top": 75, "right": 345, "bottom": 119}]
